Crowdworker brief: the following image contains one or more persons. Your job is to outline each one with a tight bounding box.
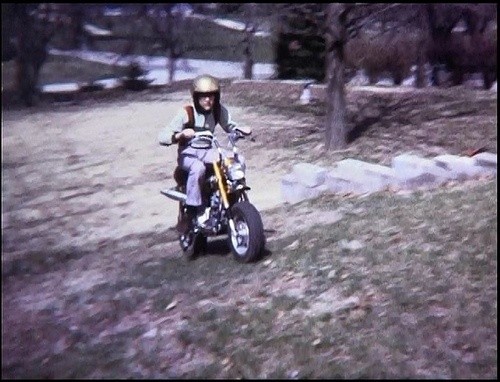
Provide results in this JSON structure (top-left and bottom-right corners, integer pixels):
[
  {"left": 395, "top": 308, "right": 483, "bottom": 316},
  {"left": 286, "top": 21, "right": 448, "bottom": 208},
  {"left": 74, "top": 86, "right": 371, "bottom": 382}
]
[{"left": 158, "top": 75, "right": 247, "bottom": 233}]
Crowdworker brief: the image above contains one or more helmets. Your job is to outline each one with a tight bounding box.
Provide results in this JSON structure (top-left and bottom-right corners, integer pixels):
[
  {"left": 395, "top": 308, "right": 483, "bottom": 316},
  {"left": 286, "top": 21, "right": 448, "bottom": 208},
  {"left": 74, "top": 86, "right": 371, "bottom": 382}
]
[{"left": 190, "top": 74, "right": 220, "bottom": 114}]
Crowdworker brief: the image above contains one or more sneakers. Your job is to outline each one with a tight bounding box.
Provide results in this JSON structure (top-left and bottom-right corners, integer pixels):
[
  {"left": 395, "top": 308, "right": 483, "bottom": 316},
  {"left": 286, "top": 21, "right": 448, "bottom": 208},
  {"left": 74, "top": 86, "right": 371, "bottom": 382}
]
[{"left": 177, "top": 205, "right": 194, "bottom": 233}]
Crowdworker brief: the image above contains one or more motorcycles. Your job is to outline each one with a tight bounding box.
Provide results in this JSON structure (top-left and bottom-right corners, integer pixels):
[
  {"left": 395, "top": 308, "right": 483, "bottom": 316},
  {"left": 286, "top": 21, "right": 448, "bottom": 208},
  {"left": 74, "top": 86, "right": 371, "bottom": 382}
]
[{"left": 159, "top": 130, "right": 264, "bottom": 264}]
